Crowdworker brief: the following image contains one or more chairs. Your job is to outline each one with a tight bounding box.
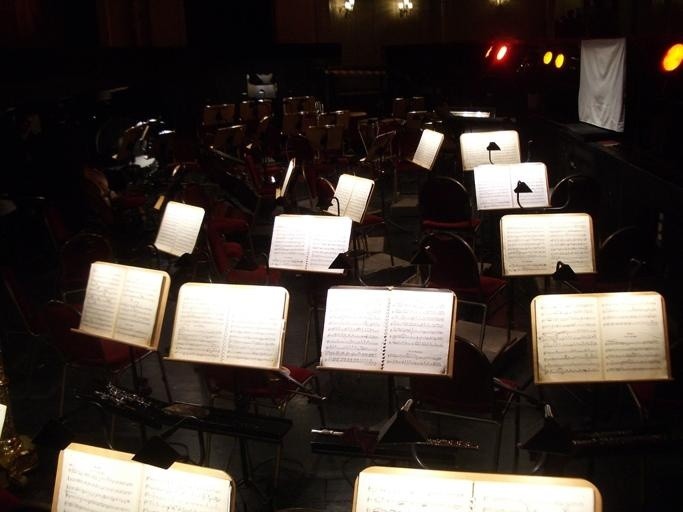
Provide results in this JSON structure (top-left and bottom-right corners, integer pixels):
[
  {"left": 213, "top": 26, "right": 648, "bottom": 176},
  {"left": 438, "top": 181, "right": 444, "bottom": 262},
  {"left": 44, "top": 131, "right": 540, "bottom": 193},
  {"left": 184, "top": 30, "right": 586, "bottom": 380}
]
[{"left": 2, "top": 90, "right": 680, "bottom": 511}]
